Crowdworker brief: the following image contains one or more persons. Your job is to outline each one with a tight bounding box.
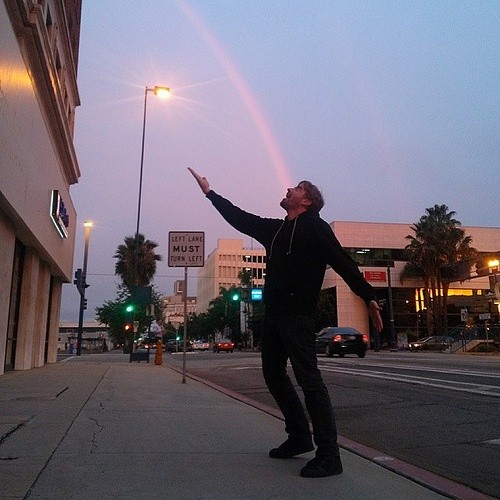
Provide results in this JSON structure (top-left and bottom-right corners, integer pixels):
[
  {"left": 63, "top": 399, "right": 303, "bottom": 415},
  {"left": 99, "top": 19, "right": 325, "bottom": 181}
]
[
  {"left": 187, "top": 167, "right": 383, "bottom": 478},
  {"left": 153, "top": 318, "right": 165, "bottom": 345}
]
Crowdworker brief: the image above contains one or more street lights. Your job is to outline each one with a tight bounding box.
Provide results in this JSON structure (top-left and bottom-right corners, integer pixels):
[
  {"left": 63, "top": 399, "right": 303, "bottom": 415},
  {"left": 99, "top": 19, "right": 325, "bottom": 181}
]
[
  {"left": 74, "top": 220, "right": 93, "bottom": 355},
  {"left": 132, "top": 85, "right": 171, "bottom": 284}
]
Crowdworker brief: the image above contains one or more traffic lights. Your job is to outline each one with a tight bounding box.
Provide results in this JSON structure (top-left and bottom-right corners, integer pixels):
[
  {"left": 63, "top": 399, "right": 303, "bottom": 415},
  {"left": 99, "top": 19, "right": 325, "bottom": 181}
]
[
  {"left": 125, "top": 304, "right": 134, "bottom": 314},
  {"left": 232, "top": 292, "right": 241, "bottom": 303},
  {"left": 124, "top": 324, "right": 132, "bottom": 332},
  {"left": 176, "top": 336, "right": 181, "bottom": 341}
]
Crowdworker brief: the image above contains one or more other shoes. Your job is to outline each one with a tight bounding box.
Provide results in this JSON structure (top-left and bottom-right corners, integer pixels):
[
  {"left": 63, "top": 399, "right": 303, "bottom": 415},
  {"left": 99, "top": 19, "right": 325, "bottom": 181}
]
[
  {"left": 268, "top": 437, "right": 316, "bottom": 459},
  {"left": 300, "top": 457, "right": 343, "bottom": 479}
]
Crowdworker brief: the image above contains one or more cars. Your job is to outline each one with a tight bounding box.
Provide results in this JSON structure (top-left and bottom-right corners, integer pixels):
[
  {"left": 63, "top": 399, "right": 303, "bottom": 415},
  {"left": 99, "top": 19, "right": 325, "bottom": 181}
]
[
  {"left": 314, "top": 327, "right": 371, "bottom": 358},
  {"left": 215, "top": 338, "right": 235, "bottom": 354},
  {"left": 190, "top": 338, "right": 212, "bottom": 350},
  {"left": 409, "top": 336, "right": 460, "bottom": 353}
]
